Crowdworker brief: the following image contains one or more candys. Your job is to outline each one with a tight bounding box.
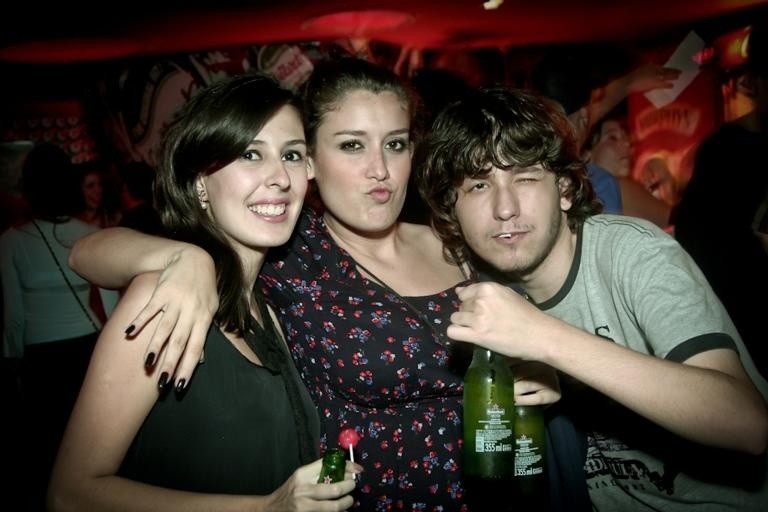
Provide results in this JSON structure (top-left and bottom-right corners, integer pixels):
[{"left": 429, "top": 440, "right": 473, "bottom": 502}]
[{"left": 339, "top": 429, "right": 359, "bottom": 479}]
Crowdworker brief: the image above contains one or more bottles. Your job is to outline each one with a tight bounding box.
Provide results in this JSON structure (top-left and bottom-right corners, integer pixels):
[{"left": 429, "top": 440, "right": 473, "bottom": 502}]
[
  {"left": 512, "top": 404, "right": 547, "bottom": 480},
  {"left": 462, "top": 344, "right": 515, "bottom": 479},
  {"left": 316, "top": 448, "right": 346, "bottom": 486}
]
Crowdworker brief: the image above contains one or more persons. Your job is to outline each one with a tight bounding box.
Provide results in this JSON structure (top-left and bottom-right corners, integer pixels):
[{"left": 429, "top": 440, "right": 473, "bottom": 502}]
[
  {"left": 412, "top": 85, "right": 768, "bottom": 512},
  {"left": 562, "top": 2, "right": 768, "bottom": 382},
  {"left": 40, "top": 69, "right": 366, "bottom": 512},
  {"left": 65, "top": 54, "right": 563, "bottom": 512},
  {"left": 1, "top": 140, "right": 170, "bottom": 512}
]
